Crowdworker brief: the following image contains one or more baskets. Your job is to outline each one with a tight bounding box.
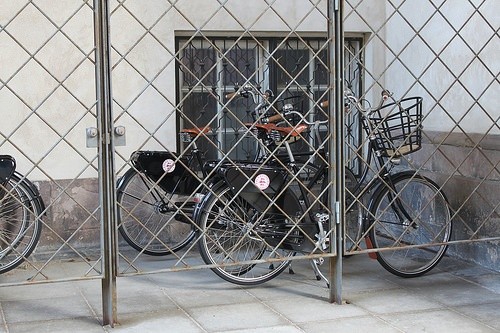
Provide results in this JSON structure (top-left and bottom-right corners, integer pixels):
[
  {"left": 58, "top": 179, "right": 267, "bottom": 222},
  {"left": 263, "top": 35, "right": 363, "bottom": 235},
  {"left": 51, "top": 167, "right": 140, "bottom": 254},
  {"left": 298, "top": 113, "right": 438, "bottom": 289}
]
[
  {"left": 250, "top": 93, "right": 305, "bottom": 147},
  {"left": 360, "top": 94, "right": 423, "bottom": 158}
]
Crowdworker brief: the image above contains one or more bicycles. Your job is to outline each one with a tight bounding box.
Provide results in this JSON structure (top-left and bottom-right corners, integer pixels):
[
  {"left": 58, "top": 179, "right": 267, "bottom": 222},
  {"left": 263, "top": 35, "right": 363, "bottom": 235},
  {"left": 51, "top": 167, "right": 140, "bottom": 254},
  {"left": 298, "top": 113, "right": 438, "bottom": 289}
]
[
  {"left": 116, "top": 87, "right": 364, "bottom": 274},
  {"left": 0, "top": 155, "right": 47, "bottom": 273},
  {"left": 189, "top": 88, "right": 452, "bottom": 285}
]
